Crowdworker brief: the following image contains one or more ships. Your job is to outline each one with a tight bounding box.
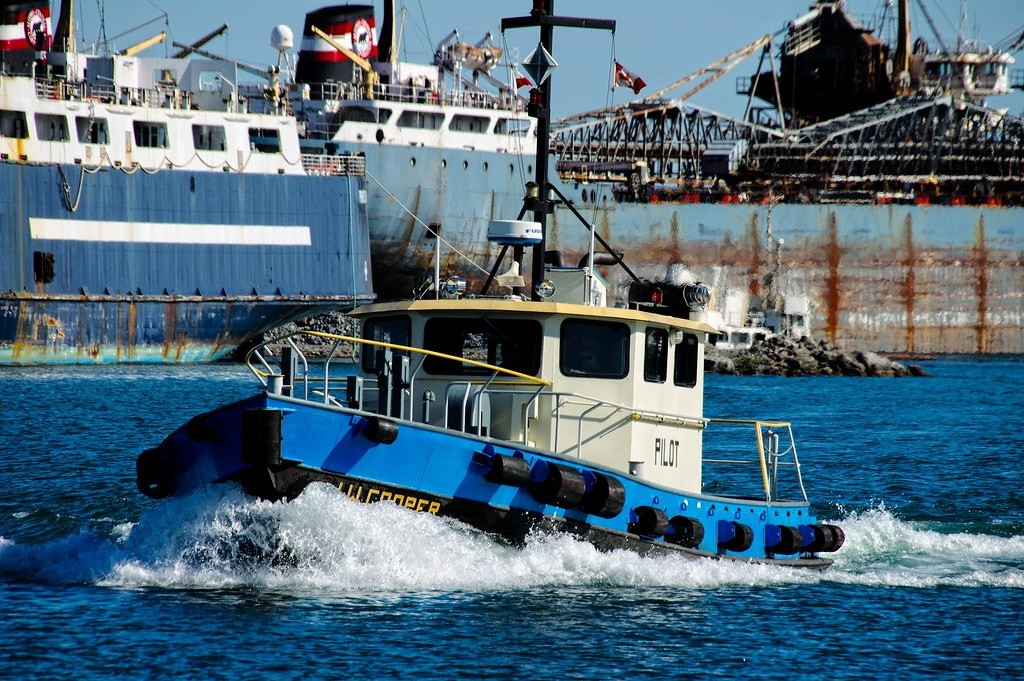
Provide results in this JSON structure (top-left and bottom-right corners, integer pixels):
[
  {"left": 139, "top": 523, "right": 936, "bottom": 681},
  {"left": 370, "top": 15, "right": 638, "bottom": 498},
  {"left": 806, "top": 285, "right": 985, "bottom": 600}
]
[
  {"left": 253, "top": 0, "right": 1024, "bottom": 360},
  {"left": 1, "top": 0, "right": 380, "bottom": 367}
]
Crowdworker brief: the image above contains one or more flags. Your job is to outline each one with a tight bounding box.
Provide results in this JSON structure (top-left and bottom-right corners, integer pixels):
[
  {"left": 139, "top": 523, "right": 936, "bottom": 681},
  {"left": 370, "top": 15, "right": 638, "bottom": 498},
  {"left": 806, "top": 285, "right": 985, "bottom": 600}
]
[
  {"left": 613, "top": 61, "right": 646, "bottom": 94},
  {"left": 513, "top": 70, "right": 532, "bottom": 99}
]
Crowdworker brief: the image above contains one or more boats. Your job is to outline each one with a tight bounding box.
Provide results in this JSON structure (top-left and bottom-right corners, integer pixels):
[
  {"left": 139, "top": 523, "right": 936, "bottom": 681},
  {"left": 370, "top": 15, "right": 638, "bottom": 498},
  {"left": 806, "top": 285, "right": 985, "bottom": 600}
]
[{"left": 135, "top": 0, "right": 846, "bottom": 578}]
